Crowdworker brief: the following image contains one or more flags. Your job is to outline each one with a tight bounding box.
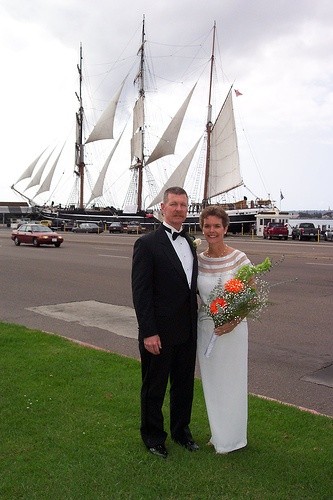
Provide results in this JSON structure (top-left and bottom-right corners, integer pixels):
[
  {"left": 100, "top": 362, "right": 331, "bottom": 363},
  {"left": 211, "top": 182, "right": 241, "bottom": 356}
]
[
  {"left": 235, "top": 90, "right": 243, "bottom": 97},
  {"left": 280, "top": 191, "right": 284, "bottom": 200}
]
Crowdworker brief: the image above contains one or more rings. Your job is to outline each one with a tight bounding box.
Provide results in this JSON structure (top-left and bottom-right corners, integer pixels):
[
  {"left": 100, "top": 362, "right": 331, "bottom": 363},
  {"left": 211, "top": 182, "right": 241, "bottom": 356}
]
[{"left": 223, "top": 331, "right": 225, "bottom": 333}]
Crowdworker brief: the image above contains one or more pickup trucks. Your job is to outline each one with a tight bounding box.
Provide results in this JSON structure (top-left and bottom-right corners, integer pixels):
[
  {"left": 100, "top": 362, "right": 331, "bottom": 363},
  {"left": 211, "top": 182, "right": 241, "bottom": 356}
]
[
  {"left": 291, "top": 223, "right": 321, "bottom": 241},
  {"left": 263, "top": 222, "right": 289, "bottom": 240}
]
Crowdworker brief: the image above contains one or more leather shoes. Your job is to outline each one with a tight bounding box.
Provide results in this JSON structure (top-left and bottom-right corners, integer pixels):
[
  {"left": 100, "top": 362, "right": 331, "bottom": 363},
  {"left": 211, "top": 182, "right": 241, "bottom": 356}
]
[
  {"left": 149, "top": 443, "right": 168, "bottom": 458},
  {"left": 175, "top": 439, "right": 200, "bottom": 451}
]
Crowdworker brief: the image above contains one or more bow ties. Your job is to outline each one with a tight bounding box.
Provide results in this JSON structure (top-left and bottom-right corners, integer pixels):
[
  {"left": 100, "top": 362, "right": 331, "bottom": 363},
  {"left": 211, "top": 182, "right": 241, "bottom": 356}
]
[{"left": 163, "top": 225, "right": 188, "bottom": 241}]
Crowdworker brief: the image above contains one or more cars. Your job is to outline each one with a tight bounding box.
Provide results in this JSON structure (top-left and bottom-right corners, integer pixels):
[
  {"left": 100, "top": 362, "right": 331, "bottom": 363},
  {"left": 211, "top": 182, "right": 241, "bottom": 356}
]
[
  {"left": 10, "top": 223, "right": 64, "bottom": 247},
  {"left": 72, "top": 223, "right": 104, "bottom": 234},
  {"left": 109, "top": 222, "right": 124, "bottom": 234},
  {"left": 127, "top": 221, "right": 143, "bottom": 234},
  {"left": 324, "top": 226, "right": 333, "bottom": 242}
]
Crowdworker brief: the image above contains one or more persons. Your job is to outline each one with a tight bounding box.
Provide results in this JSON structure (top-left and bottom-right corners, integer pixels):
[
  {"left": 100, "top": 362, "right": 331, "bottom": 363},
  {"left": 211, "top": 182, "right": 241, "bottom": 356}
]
[
  {"left": 131, "top": 187, "right": 199, "bottom": 458},
  {"left": 197, "top": 206, "right": 260, "bottom": 454}
]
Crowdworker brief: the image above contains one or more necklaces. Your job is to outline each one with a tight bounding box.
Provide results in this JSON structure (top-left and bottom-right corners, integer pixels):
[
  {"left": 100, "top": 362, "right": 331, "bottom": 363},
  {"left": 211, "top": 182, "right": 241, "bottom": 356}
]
[{"left": 206, "top": 244, "right": 227, "bottom": 258}]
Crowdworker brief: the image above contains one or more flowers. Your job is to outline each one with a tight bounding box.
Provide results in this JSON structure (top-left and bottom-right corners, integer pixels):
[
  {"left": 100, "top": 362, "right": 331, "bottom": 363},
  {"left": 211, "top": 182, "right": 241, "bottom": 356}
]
[
  {"left": 193, "top": 238, "right": 201, "bottom": 248},
  {"left": 200, "top": 254, "right": 272, "bottom": 357}
]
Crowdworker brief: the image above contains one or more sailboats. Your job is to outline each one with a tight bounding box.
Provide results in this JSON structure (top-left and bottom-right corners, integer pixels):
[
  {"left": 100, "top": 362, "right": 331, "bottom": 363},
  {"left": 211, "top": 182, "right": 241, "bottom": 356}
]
[{"left": 39, "top": 11, "right": 282, "bottom": 236}]
